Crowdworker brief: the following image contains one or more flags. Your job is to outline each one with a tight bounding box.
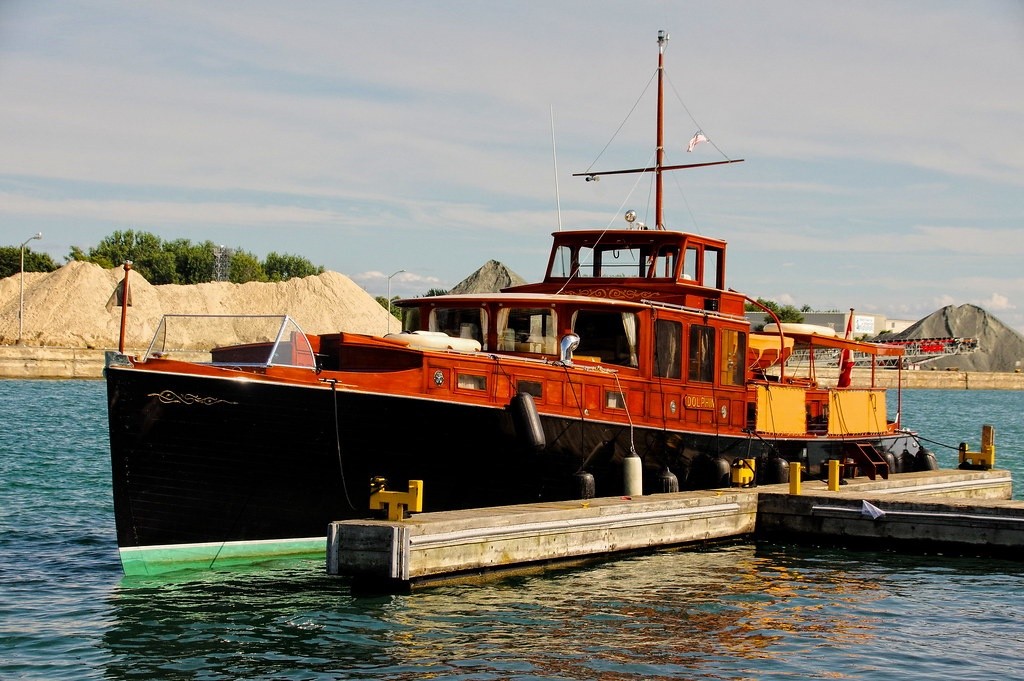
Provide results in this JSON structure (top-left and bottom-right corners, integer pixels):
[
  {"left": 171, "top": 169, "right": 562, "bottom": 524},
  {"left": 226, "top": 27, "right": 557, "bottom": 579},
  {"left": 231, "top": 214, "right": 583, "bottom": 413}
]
[
  {"left": 837, "top": 314, "right": 855, "bottom": 389},
  {"left": 686, "top": 129, "right": 710, "bottom": 153}
]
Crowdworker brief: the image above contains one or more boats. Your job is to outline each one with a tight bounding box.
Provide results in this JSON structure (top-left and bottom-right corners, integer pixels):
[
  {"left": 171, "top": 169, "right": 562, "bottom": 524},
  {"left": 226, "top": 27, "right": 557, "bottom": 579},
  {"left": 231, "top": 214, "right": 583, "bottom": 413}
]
[{"left": 100, "top": 27, "right": 983, "bottom": 595}]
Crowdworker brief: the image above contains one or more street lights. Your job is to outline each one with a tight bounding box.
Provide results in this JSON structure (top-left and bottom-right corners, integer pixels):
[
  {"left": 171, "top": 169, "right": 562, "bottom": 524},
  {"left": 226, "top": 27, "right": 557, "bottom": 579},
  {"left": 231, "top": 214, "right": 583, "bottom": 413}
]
[
  {"left": 386, "top": 269, "right": 406, "bottom": 333},
  {"left": 19, "top": 232, "right": 43, "bottom": 343}
]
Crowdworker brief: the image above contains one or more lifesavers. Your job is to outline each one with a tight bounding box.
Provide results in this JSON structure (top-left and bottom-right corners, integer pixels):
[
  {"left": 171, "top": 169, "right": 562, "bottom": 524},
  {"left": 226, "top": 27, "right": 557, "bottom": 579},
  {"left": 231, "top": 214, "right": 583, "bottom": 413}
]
[
  {"left": 387, "top": 330, "right": 482, "bottom": 354},
  {"left": 763, "top": 322, "right": 836, "bottom": 336}
]
